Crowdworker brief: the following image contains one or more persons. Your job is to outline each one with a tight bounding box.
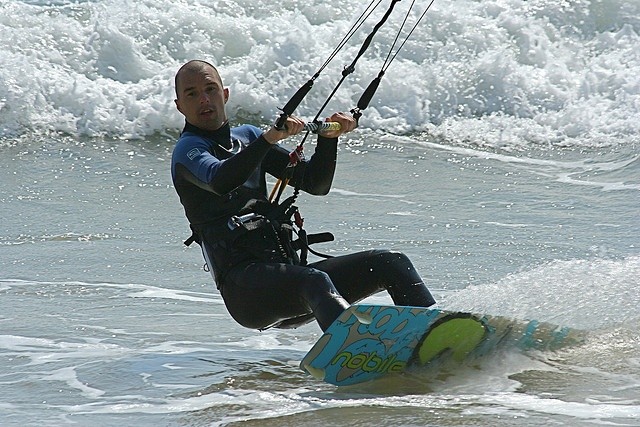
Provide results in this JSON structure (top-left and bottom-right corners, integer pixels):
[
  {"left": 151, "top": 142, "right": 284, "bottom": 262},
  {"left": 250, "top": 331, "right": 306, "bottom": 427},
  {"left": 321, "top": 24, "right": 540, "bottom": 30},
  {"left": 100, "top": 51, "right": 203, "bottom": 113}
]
[{"left": 170, "top": 59, "right": 440, "bottom": 334}]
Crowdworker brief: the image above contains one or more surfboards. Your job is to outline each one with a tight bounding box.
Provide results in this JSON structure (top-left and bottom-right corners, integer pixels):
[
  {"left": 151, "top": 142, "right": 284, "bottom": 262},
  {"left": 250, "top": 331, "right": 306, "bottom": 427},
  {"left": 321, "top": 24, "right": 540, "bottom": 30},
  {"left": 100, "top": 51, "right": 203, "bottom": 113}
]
[{"left": 299, "top": 304, "right": 590, "bottom": 385}]
